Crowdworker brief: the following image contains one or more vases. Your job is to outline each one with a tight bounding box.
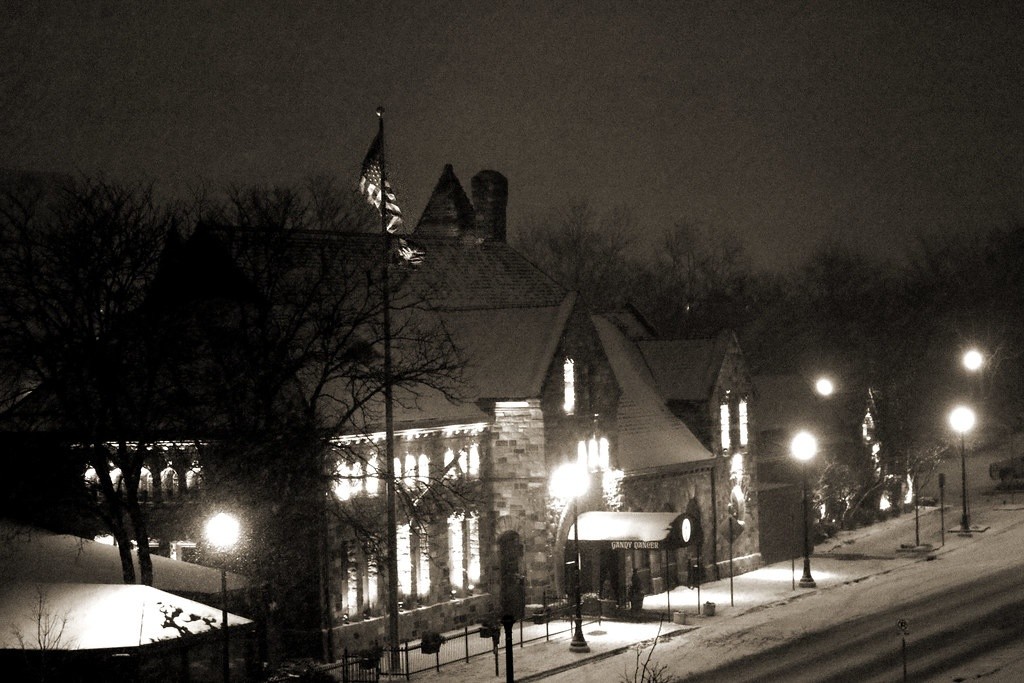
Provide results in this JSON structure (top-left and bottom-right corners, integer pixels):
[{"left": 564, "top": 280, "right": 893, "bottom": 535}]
[{"left": 532, "top": 609, "right": 545, "bottom": 625}]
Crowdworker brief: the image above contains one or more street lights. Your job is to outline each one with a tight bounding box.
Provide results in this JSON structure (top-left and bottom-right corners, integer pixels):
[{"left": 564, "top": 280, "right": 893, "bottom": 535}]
[
  {"left": 789, "top": 430, "right": 819, "bottom": 588},
  {"left": 550, "top": 464, "right": 591, "bottom": 654},
  {"left": 948, "top": 404, "right": 976, "bottom": 531}
]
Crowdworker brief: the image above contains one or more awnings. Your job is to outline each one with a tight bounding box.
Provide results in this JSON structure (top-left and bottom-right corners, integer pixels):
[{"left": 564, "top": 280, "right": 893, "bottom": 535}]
[{"left": 568, "top": 510, "right": 707, "bottom": 623}]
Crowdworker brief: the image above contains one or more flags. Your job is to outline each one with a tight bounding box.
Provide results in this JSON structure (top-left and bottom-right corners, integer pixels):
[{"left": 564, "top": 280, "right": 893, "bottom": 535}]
[{"left": 359, "top": 125, "right": 404, "bottom": 235}]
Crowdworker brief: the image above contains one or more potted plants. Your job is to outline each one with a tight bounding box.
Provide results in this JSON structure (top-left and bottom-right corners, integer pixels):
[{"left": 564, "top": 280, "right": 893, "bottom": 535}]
[
  {"left": 626, "top": 569, "right": 644, "bottom": 609},
  {"left": 420, "top": 630, "right": 448, "bottom": 654},
  {"left": 359, "top": 646, "right": 383, "bottom": 670},
  {"left": 703, "top": 601, "right": 715, "bottom": 616},
  {"left": 478, "top": 616, "right": 491, "bottom": 638},
  {"left": 672, "top": 609, "right": 687, "bottom": 623},
  {"left": 597, "top": 580, "right": 618, "bottom": 615}
]
